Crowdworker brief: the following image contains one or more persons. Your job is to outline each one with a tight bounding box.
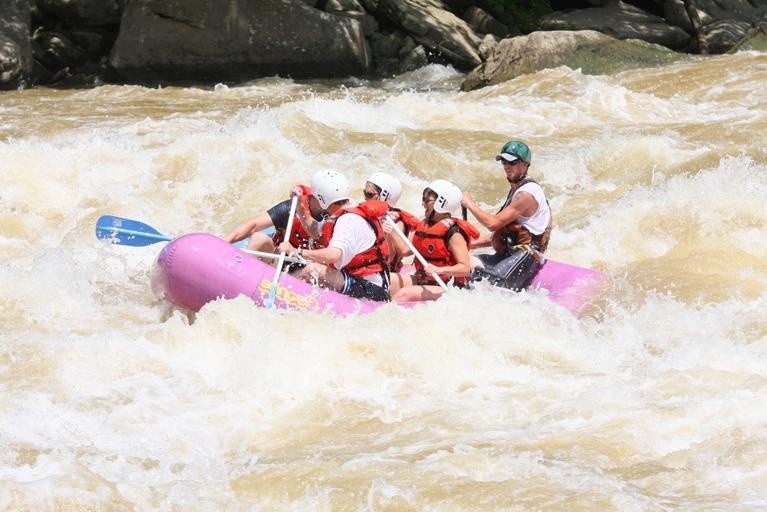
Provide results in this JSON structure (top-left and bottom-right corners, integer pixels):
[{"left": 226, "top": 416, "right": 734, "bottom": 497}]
[
  {"left": 460, "top": 141, "right": 552, "bottom": 294},
  {"left": 225, "top": 170, "right": 390, "bottom": 302},
  {"left": 362, "top": 173, "right": 473, "bottom": 303}
]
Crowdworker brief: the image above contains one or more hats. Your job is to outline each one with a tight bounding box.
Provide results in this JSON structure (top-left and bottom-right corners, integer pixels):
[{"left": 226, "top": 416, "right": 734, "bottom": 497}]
[{"left": 496, "top": 141, "right": 531, "bottom": 164}]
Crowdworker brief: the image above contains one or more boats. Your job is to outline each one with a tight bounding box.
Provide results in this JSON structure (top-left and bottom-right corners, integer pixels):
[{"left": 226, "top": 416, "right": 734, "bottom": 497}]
[{"left": 151, "top": 228, "right": 609, "bottom": 323}]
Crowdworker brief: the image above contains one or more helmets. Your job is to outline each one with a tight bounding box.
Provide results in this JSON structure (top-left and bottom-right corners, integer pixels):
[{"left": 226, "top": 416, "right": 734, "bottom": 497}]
[
  {"left": 424, "top": 179, "right": 462, "bottom": 214},
  {"left": 367, "top": 172, "right": 400, "bottom": 207},
  {"left": 312, "top": 170, "right": 351, "bottom": 208}
]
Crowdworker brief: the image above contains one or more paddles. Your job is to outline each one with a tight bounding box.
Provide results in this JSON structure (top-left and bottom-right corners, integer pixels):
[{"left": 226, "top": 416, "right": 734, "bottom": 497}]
[{"left": 95, "top": 215, "right": 312, "bottom": 266}]
